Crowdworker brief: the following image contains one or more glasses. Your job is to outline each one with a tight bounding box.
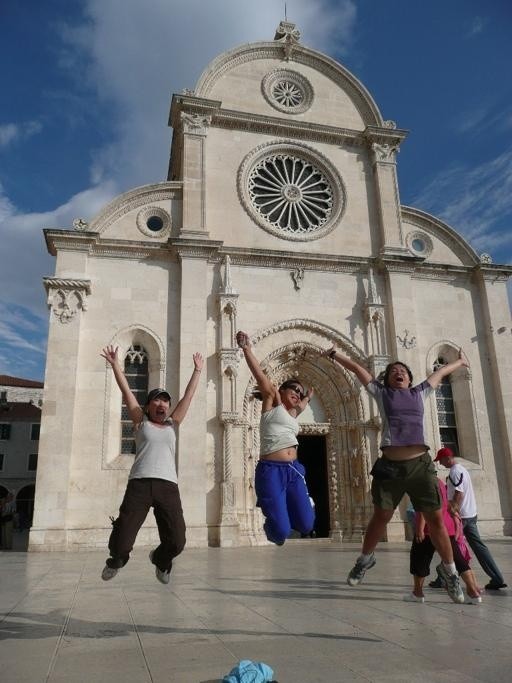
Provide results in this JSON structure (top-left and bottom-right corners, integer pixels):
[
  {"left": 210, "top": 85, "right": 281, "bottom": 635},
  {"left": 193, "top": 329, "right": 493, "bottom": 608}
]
[{"left": 287, "top": 384, "right": 306, "bottom": 402}]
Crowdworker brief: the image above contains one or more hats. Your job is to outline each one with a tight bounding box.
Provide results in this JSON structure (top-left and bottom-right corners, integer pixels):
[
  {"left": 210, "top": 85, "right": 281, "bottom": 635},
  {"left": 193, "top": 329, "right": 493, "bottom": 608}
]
[
  {"left": 147, "top": 387, "right": 173, "bottom": 404},
  {"left": 432, "top": 447, "right": 454, "bottom": 462}
]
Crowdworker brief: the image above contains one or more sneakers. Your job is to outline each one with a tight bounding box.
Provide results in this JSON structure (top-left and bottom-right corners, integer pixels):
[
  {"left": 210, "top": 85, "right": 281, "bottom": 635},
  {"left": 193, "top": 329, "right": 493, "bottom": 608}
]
[
  {"left": 148, "top": 549, "right": 170, "bottom": 584},
  {"left": 346, "top": 551, "right": 377, "bottom": 587},
  {"left": 402, "top": 591, "right": 424, "bottom": 602},
  {"left": 101, "top": 564, "right": 118, "bottom": 581},
  {"left": 435, "top": 560, "right": 465, "bottom": 604}
]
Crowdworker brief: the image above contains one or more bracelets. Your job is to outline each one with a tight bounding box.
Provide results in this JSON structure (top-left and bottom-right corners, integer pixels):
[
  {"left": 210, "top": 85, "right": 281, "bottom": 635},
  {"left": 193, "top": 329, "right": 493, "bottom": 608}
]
[
  {"left": 194, "top": 368, "right": 202, "bottom": 373},
  {"left": 304, "top": 396, "right": 311, "bottom": 403},
  {"left": 330, "top": 350, "right": 336, "bottom": 359}
]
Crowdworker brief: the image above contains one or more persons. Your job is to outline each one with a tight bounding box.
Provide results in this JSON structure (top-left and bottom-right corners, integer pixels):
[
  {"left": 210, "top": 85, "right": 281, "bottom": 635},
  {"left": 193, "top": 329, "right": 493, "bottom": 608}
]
[
  {"left": 237, "top": 330, "right": 317, "bottom": 546},
  {"left": 0, "top": 493, "right": 16, "bottom": 547},
  {"left": 428, "top": 447, "right": 507, "bottom": 589},
  {"left": 101, "top": 344, "right": 205, "bottom": 584},
  {"left": 319, "top": 342, "right": 470, "bottom": 603},
  {"left": 444, "top": 499, "right": 480, "bottom": 593},
  {"left": 409, "top": 476, "right": 482, "bottom": 604}
]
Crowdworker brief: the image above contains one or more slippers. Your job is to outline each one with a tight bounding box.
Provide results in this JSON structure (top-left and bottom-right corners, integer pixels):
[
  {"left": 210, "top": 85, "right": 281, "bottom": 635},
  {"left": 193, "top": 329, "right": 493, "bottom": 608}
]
[
  {"left": 465, "top": 589, "right": 482, "bottom": 604},
  {"left": 485, "top": 581, "right": 508, "bottom": 589},
  {"left": 429, "top": 580, "right": 441, "bottom": 588}
]
